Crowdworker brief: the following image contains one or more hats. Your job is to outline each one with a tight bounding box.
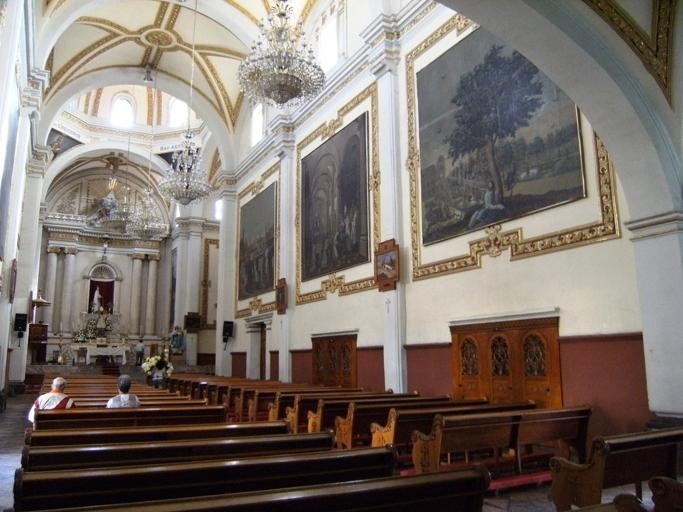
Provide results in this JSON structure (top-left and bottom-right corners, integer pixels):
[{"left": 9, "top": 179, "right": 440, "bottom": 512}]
[
  {"left": 117, "top": 374, "right": 131, "bottom": 388},
  {"left": 52, "top": 377, "right": 66, "bottom": 387}
]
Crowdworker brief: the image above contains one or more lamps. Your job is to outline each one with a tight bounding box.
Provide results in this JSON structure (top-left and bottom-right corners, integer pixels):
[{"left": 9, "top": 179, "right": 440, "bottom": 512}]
[
  {"left": 31, "top": 288, "right": 51, "bottom": 310},
  {"left": 236, "top": 0, "right": 326, "bottom": 109},
  {"left": 93, "top": 0, "right": 213, "bottom": 242}
]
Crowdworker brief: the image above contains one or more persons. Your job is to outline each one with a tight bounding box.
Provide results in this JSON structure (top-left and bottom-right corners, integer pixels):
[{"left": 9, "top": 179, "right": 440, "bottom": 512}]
[
  {"left": 238, "top": 243, "right": 271, "bottom": 296},
  {"left": 484, "top": 180, "right": 507, "bottom": 213},
  {"left": 133, "top": 340, "right": 144, "bottom": 366},
  {"left": 105, "top": 374, "right": 139, "bottom": 408},
  {"left": 307, "top": 202, "right": 358, "bottom": 274},
  {"left": 151, "top": 360, "right": 165, "bottom": 385},
  {"left": 27, "top": 376, "right": 76, "bottom": 423}
]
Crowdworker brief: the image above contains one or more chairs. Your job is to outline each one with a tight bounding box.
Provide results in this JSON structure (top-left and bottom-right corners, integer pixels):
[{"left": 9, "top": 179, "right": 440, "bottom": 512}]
[{"left": 13, "top": 371, "right": 492, "bottom": 510}]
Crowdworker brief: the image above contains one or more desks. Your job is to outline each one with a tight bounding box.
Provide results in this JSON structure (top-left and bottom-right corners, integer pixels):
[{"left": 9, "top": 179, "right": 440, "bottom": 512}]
[
  {"left": 545, "top": 426, "right": 682, "bottom": 509},
  {"left": 409, "top": 404, "right": 592, "bottom": 482},
  {"left": 71, "top": 345, "right": 127, "bottom": 366},
  {"left": 369, "top": 401, "right": 538, "bottom": 456}
]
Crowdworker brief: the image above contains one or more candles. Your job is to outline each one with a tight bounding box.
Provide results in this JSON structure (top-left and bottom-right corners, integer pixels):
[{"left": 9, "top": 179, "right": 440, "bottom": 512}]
[{"left": 60, "top": 320, "right": 63, "bottom": 332}]
